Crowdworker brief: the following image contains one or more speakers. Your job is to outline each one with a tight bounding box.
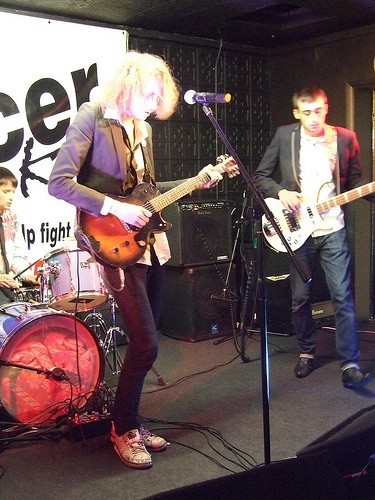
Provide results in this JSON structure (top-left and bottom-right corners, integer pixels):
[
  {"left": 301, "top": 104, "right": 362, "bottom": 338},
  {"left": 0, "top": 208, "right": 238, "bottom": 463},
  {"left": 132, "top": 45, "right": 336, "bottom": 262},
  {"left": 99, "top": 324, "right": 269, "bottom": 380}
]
[
  {"left": 140, "top": 402, "right": 375, "bottom": 500},
  {"left": 155, "top": 263, "right": 238, "bottom": 343},
  {"left": 161, "top": 197, "right": 233, "bottom": 268}
]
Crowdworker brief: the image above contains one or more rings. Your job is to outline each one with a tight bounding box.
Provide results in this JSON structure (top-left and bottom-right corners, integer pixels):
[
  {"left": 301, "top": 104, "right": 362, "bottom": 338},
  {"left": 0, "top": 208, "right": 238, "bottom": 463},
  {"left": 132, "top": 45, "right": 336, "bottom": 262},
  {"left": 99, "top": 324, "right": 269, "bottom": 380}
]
[{"left": 216, "top": 180, "right": 219, "bottom": 183}]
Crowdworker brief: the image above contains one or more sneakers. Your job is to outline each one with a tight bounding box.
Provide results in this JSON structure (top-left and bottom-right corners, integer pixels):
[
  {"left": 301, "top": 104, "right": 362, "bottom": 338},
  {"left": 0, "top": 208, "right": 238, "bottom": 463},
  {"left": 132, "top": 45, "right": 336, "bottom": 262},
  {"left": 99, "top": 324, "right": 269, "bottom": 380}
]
[
  {"left": 137, "top": 423, "right": 167, "bottom": 451},
  {"left": 110, "top": 421, "right": 153, "bottom": 469}
]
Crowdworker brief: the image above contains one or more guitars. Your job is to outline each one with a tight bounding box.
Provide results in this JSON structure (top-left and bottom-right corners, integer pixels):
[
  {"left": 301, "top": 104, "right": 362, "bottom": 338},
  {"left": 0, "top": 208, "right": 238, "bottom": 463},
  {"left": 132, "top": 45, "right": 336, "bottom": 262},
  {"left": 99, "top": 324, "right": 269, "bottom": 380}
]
[
  {"left": 262, "top": 180, "right": 375, "bottom": 253},
  {"left": 77, "top": 156, "right": 239, "bottom": 268}
]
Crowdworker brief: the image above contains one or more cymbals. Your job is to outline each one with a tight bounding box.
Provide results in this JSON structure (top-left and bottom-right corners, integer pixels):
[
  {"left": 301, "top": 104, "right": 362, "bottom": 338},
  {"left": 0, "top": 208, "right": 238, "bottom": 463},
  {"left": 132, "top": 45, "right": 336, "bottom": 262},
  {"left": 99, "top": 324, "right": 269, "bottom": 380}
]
[{"left": 63, "top": 235, "right": 77, "bottom": 242}]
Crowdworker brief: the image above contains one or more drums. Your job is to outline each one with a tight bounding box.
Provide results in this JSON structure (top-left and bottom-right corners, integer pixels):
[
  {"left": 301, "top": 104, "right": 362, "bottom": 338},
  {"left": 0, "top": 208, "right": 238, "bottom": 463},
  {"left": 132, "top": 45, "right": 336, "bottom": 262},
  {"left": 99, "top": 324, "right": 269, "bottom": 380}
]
[
  {"left": 14, "top": 286, "right": 42, "bottom": 303},
  {"left": 42, "top": 246, "right": 111, "bottom": 312},
  {"left": 2, "top": 307, "right": 104, "bottom": 430}
]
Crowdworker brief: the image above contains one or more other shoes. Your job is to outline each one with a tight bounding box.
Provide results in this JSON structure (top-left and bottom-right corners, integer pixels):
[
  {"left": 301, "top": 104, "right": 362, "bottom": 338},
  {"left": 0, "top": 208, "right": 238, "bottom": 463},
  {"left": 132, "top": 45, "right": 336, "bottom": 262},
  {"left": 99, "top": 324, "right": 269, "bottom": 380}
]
[
  {"left": 295, "top": 357, "right": 314, "bottom": 377},
  {"left": 342, "top": 368, "right": 370, "bottom": 388}
]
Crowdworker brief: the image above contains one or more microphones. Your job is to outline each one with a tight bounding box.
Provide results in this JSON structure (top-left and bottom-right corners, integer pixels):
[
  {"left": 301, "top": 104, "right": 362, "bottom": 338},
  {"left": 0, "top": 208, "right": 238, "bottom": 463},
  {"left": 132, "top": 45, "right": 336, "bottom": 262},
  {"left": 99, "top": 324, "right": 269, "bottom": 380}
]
[
  {"left": 51, "top": 368, "right": 70, "bottom": 381},
  {"left": 210, "top": 295, "right": 238, "bottom": 301},
  {"left": 183, "top": 89, "right": 231, "bottom": 105}
]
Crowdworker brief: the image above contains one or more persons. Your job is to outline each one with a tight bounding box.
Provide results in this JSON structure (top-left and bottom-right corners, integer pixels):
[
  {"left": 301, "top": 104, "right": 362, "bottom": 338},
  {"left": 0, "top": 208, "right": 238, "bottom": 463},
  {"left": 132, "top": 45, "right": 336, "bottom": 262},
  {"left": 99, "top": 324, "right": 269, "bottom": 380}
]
[
  {"left": 48, "top": 50, "right": 222, "bottom": 468},
  {"left": 0, "top": 167, "right": 45, "bottom": 305},
  {"left": 253, "top": 84, "right": 375, "bottom": 388}
]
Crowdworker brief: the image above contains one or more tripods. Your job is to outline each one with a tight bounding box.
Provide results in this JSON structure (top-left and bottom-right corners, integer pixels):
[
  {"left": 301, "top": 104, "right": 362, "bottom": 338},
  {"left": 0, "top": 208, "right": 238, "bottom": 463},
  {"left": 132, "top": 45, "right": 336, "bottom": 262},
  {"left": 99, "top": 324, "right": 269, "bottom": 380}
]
[{"left": 82, "top": 290, "right": 166, "bottom": 389}]
